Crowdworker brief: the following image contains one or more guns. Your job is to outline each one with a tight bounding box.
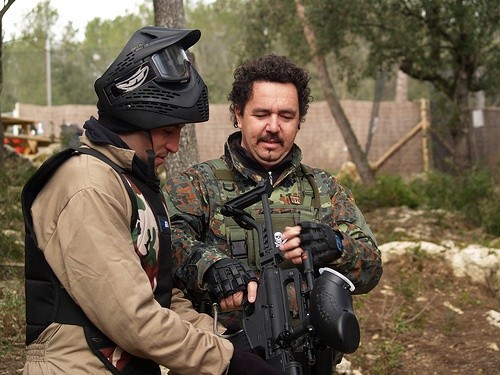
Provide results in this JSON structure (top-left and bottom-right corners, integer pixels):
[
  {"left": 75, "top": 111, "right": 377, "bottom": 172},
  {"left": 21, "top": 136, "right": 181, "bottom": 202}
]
[{"left": 220, "top": 178, "right": 362, "bottom": 375}]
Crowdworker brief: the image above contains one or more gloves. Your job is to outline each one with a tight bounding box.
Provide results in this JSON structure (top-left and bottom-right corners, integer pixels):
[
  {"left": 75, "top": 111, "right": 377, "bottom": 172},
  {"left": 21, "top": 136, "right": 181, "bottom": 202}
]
[
  {"left": 222, "top": 348, "right": 280, "bottom": 375},
  {"left": 222, "top": 322, "right": 241, "bottom": 335}
]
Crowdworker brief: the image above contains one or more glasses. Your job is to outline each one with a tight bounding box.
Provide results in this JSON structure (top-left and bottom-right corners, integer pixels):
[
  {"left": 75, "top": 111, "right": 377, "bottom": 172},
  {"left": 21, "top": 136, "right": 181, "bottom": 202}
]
[{"left": 148, "top": 40, "right": 192, "bottom": 85}]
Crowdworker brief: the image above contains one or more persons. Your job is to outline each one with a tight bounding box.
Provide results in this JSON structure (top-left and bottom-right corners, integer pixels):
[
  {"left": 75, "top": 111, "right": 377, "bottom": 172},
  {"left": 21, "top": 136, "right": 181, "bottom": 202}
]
[{"left": 21, "top": 26, "right": 383, "bottom": 375}]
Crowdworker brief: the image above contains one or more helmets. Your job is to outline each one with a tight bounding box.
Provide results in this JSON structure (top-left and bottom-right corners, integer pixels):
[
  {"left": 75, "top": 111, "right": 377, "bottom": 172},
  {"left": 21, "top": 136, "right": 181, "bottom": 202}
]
[{"left": 94, "top": 27, "right": 210, "bottom": 132}]
